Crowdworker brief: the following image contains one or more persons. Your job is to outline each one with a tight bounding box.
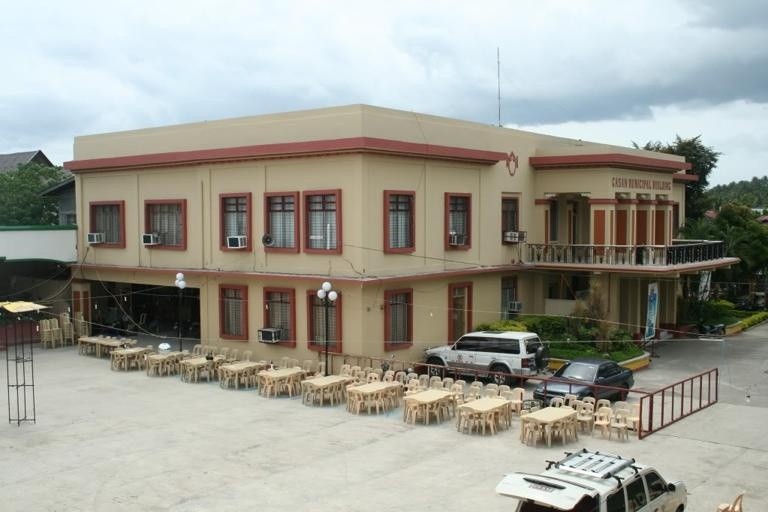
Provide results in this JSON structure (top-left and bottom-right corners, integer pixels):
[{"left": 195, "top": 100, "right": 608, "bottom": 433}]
[{"left": 121, "top": 310, "right": 129, "bottom": 335}]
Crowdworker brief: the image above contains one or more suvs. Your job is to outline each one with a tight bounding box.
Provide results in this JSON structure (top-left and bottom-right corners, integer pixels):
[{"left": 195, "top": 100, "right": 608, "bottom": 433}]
[
  {"left": 423, "top": 330, "right": 551, "bottom": 388},
  {"left": 493, "top": 447, "right": 687, "bottom": 512}
]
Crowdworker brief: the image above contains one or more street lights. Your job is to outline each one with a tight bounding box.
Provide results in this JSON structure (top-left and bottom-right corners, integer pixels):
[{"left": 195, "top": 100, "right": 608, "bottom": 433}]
[
  {"left": 316, "top": 280, "right": 338, "bottom": 374},
  {"left": 172, "top": 273, "right": 188, "bottom": 352}
]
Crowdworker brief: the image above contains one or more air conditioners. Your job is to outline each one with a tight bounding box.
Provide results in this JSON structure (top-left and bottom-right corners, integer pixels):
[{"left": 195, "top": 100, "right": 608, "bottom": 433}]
[
  {"left": 504, "top": 231, "right": 519, "bottom": 243},
  {"left": 448, "top": 231, "right": 465, "bottom": 246},
  {"left": 87, "top": 231, "right": 106, "bottom": 245},
  {"left": 226, "top": 235, "right": 248, "bottom": 249},
  {"left": 142, "top": 233, "right": 162, "bottom": 246}
]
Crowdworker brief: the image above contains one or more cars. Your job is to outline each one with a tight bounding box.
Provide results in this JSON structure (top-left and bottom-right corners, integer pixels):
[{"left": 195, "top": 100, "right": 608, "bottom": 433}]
[
  {"left": 532, "top": 356, "right": 636, "bottom": 407},
  {"left": 741, "top": 290, "right": 768, "bottom": 310}
]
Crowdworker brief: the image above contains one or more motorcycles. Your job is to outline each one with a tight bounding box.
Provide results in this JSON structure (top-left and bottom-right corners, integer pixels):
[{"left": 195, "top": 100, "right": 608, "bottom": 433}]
[{"left": 688, "top": 316, "right": 728, "bottom": 337}]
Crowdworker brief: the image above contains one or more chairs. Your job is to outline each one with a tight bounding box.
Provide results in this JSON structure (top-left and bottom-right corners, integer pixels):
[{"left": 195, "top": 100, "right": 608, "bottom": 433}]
[
  {"left": 714, "top": 491, "right": 745, "bottom": 512},
  {"left": 38, "top": 317, "right": 300, "bottom": 402},
  {"left": 301, "top": 362, "right": 645, "bottom": 450}
]
